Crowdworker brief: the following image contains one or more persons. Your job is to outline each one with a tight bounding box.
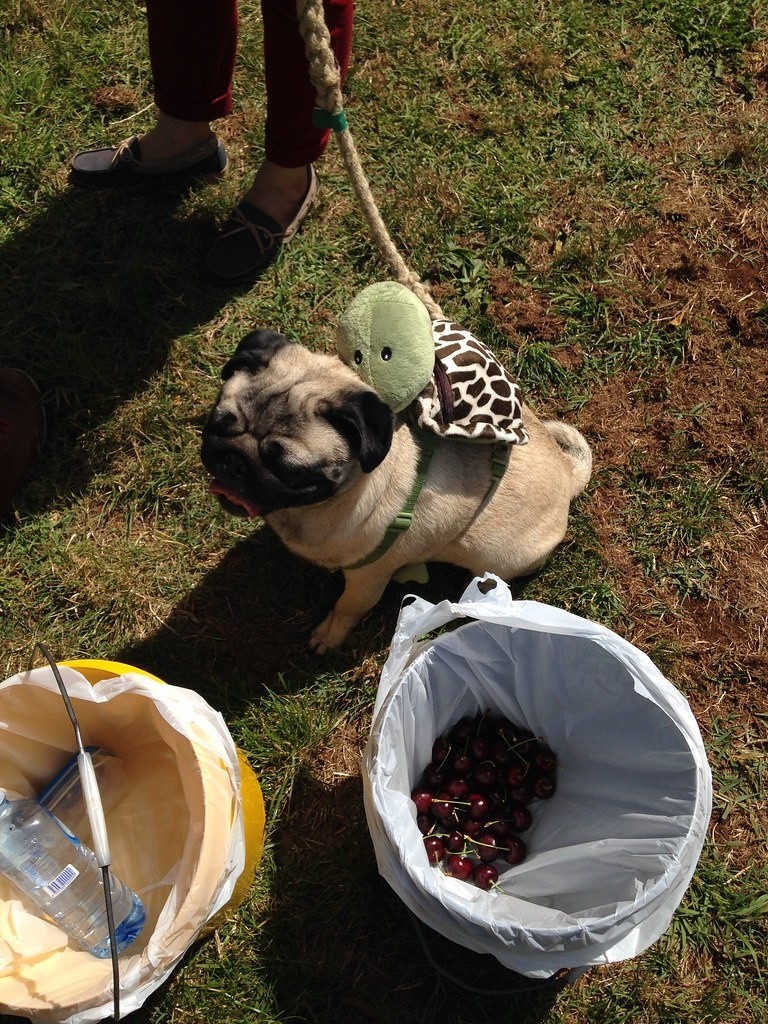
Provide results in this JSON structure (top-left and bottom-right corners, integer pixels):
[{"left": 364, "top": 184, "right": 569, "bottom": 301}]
[{"left": 70, "top": 0, "right": 353, "bottom": 281}]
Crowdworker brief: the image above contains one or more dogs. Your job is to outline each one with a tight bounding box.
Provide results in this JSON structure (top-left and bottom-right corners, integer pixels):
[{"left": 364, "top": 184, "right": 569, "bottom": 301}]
[{"left": 199, "top": 330, "right": 593, "bottom": 658}]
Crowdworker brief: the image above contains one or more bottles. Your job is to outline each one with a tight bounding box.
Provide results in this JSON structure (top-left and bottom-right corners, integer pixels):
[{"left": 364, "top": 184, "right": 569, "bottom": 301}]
[{"left": 0, "top": 787, "right": 146, "bottom": 959}]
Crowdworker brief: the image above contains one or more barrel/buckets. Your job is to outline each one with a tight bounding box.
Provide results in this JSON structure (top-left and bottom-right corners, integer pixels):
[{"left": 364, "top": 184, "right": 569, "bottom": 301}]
[
  {"left": 373, "top": 620, "right": 713, "bottom": 996},
  {"left": 0, "top": 643, "right": 267, "bottom": 1024}
]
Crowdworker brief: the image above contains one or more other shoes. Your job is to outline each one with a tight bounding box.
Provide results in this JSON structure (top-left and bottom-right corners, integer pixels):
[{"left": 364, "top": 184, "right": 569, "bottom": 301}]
[
  {"left": 204, "top": 162, "right": 319, "bottom": 280},
  {"left": 71, "top": 129, "right": 228, "bottom": 193}
]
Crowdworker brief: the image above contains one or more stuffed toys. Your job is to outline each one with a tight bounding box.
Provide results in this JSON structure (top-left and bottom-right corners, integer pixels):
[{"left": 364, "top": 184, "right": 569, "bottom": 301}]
[{"left": 336, "top": 281, "right": 532, "bottom": 446}]
